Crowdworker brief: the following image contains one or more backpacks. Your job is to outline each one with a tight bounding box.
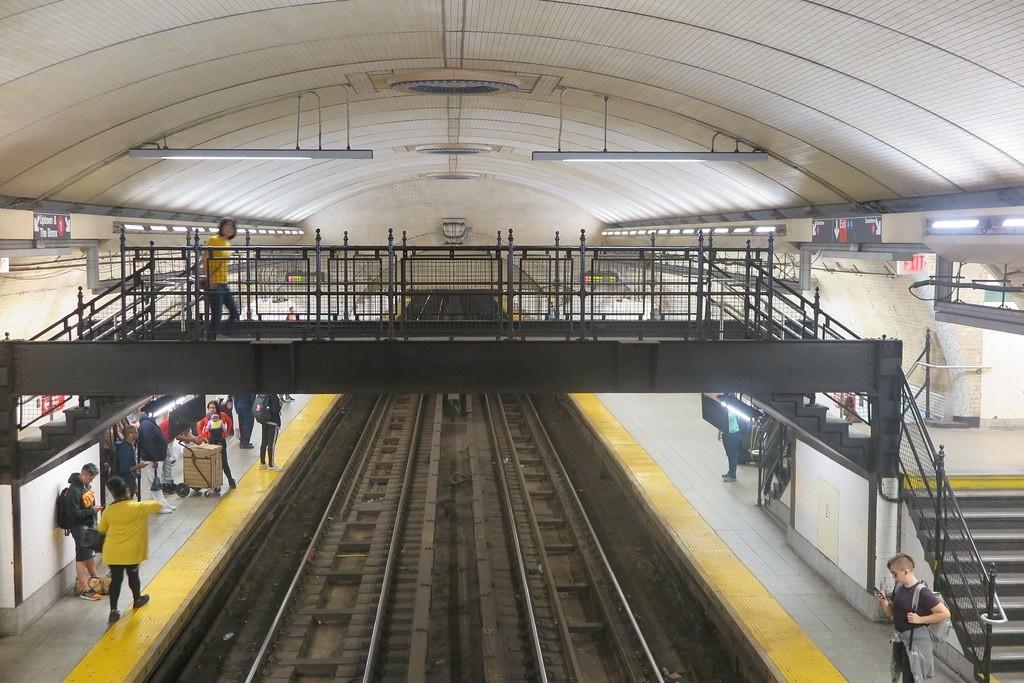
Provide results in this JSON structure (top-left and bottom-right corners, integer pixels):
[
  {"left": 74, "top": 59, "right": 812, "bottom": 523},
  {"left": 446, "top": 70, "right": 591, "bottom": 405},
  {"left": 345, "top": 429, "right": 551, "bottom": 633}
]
[
  {"left": 110, "top": 445, "right": 129, "bottom": 475},
  {"left": 252, "top": 394, "right": 273, "bottom": 423},
  {"left": 57, "top": 487, "right": 82, "bottom": 529},
  {"left": 894, "top": 582, "right": 949, "bottom": 642}
]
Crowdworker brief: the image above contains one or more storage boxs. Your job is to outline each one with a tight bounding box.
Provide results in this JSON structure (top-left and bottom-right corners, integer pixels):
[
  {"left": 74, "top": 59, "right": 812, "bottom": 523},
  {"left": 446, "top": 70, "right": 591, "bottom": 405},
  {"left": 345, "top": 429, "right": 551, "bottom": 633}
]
[{"left": 183, "top": 445, "right": 222, "bottom": 488}]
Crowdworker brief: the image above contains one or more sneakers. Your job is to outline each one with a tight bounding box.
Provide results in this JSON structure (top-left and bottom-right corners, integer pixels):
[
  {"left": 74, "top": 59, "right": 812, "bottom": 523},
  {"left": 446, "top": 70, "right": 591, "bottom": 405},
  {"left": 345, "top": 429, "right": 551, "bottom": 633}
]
[{"left": 81, "top": 588, "right": 102, "bottom": 600}]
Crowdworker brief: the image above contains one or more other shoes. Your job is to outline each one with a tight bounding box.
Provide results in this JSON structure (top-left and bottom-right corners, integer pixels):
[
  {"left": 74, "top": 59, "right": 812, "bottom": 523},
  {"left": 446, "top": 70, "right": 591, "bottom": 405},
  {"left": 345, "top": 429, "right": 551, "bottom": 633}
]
[
  {"left": 267, "top": 465, "right": 282, "bottom": 471},
  {"left": 285, "top": 396, "right": 295, "bottom": 401},
  {"left": 723, "top": 476, "right": 736, "bottom": 482},
  {"left": 168, "top": 505, "right": 177, "bottom": 510},
  {"left": 109, "top": 611, "right": 120, "bottom": 621},
  {"left": 259, "top": 462, "right": 268, "bottom": 470},
  {"left": 133, "top": 595, "right": 149, "bottom": 607},
  {"left": 249, "top": 443, "right": 253, "bottom": 445},
  {"left": 721, "top": 472, "right": 730, "bottom": 478},
  {"left": 229, "top": 480, "right": 236, "bottom": 489},
  {"left": 160, "top": 509, "right": 173, "bottom": 513},
  {"left": 241, "top": 445, "right": 254, "bottom": 449}
]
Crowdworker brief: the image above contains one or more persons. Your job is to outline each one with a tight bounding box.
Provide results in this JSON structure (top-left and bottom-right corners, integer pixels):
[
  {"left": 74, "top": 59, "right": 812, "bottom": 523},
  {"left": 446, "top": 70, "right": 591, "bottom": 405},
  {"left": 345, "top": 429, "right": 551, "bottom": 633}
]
[
  {"left": 251, "top": 393, "right": 284, "bottom": 471},
  {"left": 96, "top": 477, "right": 163, "bottom": 622},
  {"left": 140, "top": 403, "right": 177, "bottom": 514},
  {"left": 279, "top": 393, "right": 295, "bottom": 403},
  {"left": 197, "top": 401, "right": 237, "bottom": 488},
  {"left": 233, "top": 393, "right": 254, "bottom": 449},
  {"left": 66, "top": 463, "right": 104, "bottom": 601},
  {"left": 159, "top": 416, "right": 202, "bottom": 490},
  {"left": 109, "top": 425, "right": 148, "bottom": 498},
  {"left": 215, "top": 395, "right": 234, "bottom": 435},
  {"left": 874, "top": 553, "right": 951, "bottom": 683},
  {"left": 718, "top": 405, "right": 742, "bottom": 482},
  {"left": 202, "top": 217, "right": 239, "bottom": 340}
]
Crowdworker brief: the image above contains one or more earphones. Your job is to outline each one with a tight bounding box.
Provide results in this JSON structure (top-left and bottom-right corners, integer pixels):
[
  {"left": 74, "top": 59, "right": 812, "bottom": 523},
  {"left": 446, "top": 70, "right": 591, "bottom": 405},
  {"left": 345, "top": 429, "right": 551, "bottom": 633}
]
[
  {"left": 83, "top": 475, "right": 85, "bottom": 478},
  {"left": 129, "top": 434, "right": 132, "bottom": 437}
]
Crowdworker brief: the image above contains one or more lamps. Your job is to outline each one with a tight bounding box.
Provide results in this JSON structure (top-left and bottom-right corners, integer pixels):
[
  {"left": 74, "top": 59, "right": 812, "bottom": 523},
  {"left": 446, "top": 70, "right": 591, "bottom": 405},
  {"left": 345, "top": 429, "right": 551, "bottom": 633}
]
[
  {"left": 531, "top": 96, "right": 769, "bottom": 164},
  {"left": 922, "top": 214, "right": 1024, "bottom": 235},
  {"left": 717, "top": 394, "right": 763, "bottom": 422},
  {"left": 140, "top": 395, "right": 185, "bottom": 418},
  {"left": 112, "top": 221, "right": 304, "bottom": 236},
  {"left": 129, "top": 91, "right": 374, "bottom": 160},
  {"left": 601, "top": 224, "right": 786, "bottom": 236}
]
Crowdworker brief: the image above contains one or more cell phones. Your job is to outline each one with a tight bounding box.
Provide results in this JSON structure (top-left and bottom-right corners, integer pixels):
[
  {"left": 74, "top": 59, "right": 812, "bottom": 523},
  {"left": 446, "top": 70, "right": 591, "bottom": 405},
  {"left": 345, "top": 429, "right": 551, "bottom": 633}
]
[
  {"left": 872, "top": 586, "right": 887, "bottom": 600},
  {"left": 144, "top": 462, "right": 149, "bottom": 465}
]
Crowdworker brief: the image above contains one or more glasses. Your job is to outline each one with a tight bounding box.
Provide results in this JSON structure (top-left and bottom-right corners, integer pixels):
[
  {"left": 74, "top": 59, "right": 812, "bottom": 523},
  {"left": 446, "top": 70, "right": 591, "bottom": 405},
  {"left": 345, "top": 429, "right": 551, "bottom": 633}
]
[{"left": 890, "top": 571, "right": 904, "bottom": 577}]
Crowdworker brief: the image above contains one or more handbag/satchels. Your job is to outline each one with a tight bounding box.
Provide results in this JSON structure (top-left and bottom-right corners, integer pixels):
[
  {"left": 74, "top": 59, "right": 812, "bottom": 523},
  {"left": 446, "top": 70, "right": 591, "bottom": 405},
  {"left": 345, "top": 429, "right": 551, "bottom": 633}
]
[
  {"left": 75, "top": 576, "right": 112, "bottom": 595},
  {"left": 150, "top": 466, "right": 163, "bottom": 491}
]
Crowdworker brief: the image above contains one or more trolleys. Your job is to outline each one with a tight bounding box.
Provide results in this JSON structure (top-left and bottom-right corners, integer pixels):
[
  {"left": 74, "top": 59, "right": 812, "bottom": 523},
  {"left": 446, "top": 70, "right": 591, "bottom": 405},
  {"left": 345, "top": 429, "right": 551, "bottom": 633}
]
[{"left": 177, "top": 440, "right": 223, "bottom": 498}]
